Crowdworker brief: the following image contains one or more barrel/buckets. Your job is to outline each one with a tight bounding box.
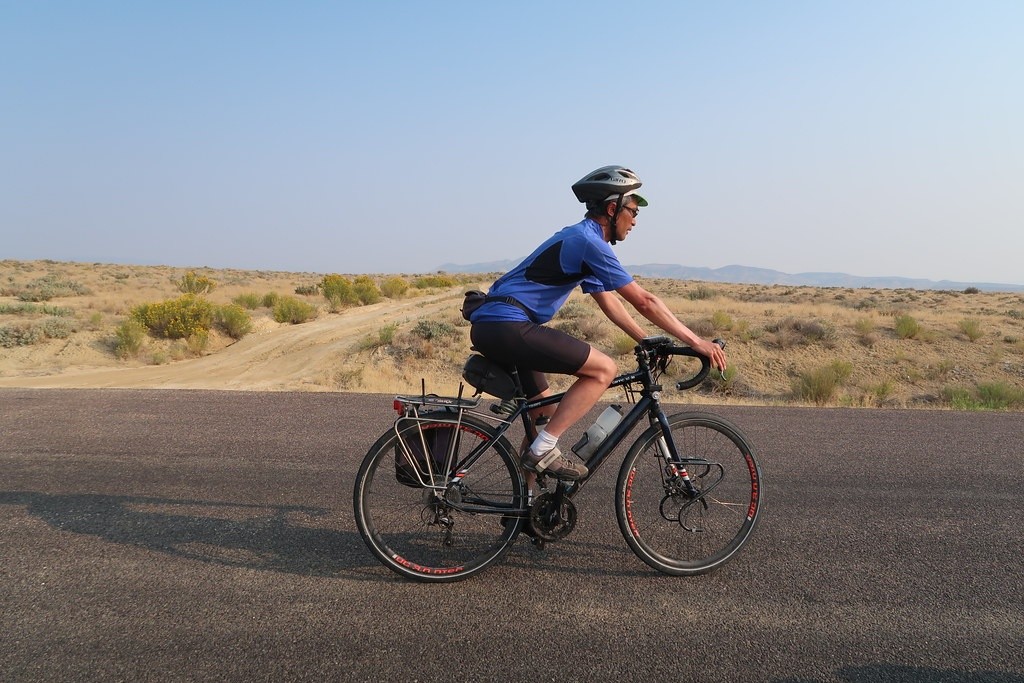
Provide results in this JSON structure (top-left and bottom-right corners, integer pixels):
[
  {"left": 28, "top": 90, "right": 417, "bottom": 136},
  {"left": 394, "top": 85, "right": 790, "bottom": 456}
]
[{"left": 392, "top": 393, "right": 462, "bottom": 488}]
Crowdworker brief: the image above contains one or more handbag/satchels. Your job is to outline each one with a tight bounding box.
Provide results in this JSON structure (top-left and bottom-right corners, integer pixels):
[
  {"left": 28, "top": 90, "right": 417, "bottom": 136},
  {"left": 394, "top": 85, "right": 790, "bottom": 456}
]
[
  {"left": 461, "top": 289, "right": 486, "bottom": 321},
  {"left": 395, "top": 393, "right": 461, "bottom": 488}
]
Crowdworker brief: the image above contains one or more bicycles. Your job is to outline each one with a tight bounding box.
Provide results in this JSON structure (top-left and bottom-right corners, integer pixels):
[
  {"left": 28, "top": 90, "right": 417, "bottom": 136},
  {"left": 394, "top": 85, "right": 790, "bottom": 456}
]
[{"left": 353, "top": 335, "right": 763, "bottom": 583}]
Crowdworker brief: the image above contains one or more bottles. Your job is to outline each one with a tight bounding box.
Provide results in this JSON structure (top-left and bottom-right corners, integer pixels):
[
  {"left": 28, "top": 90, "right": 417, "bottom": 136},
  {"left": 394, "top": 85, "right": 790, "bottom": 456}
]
[
  {"left": 532, "top": 409, "right": 552, "bottom": 436},
  {"left": 570, "top": 403, "right": 624, "bottom": 463}
]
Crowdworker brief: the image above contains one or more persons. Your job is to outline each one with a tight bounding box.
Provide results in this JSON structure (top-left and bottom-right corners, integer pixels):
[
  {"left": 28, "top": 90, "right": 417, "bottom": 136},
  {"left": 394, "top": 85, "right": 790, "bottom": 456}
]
[{"left": 469, "top": 165, "right": 727, "bottom": 509}]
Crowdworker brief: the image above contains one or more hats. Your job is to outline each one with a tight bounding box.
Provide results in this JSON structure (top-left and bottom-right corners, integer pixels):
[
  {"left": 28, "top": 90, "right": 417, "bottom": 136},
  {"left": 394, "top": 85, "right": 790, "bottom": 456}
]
[{"left": 604, "top": 189, "right": 649, "bottom": 207}]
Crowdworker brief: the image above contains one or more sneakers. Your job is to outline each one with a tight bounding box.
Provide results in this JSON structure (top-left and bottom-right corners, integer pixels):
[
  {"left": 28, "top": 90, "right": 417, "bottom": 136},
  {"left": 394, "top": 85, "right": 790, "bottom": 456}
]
[
  {"left": 500, "top": 513, "right": 539, "bottom": 538},
  {"left": 520, "top": 447, "right": 588, "bottom": 480}
]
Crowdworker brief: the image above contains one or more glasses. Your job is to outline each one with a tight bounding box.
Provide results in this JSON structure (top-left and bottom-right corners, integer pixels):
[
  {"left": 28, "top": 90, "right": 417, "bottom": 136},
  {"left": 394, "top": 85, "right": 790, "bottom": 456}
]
[{"left": 626, "top": 204, "right": 640, "bottom": 218}]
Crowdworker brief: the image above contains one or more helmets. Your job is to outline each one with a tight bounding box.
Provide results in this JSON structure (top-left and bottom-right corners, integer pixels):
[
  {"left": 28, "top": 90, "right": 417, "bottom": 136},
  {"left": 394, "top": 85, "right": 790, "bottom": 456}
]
[{"left": 572, "top": 165, "right": 642, "bottom": 203}]
